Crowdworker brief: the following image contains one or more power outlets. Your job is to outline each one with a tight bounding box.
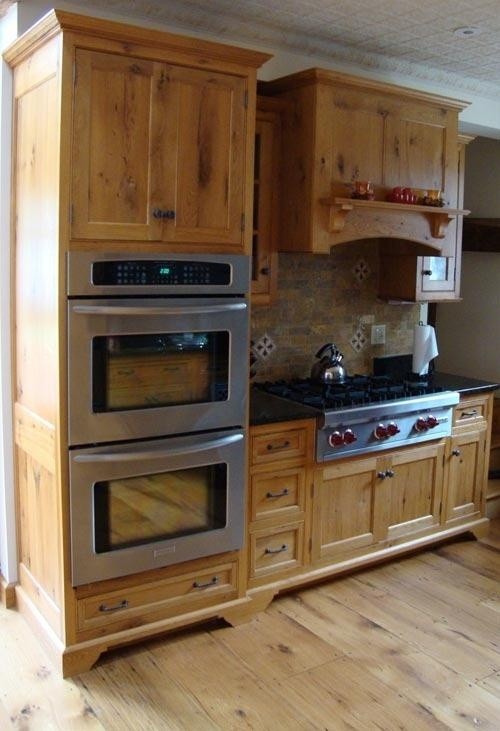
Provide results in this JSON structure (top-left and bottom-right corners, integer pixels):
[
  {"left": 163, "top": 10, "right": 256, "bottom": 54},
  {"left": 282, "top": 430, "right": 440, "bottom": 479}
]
[{"left": 370, "top": 325, "right": 386, "bottom": 345}]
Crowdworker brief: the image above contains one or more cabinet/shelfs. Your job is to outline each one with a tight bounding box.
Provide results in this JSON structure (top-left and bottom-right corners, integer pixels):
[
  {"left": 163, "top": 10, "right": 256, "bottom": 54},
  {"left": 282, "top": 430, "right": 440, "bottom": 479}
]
[
  {"left": 272, "top": 68, "right": 471, "bottom": 258},
  {"left": 250, "top": 77, "right": 279, "bottom": 305},
  {"left": 377, "top": 141, "right": 464, "bottom": 305},
  {"left": 2, "top": 7, "right": 274, "bottom": 257},
  {"left": 443, "top": 389, "right": 493, "bottom": 540},
  {"left": 312, "top": 438, "right": 444, "bottom": 582},
  {"left": 247, "top": 417, "right": 314, "bottom": 618},
  {"left": 64, "top": 548, "right": 247, "bottom": 676}
]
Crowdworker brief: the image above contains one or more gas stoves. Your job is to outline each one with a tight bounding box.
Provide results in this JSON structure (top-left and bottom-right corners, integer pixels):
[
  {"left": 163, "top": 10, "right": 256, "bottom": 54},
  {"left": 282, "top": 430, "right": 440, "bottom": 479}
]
[{"left": 255, "top": 372, "right": 459, "bottom": 411}]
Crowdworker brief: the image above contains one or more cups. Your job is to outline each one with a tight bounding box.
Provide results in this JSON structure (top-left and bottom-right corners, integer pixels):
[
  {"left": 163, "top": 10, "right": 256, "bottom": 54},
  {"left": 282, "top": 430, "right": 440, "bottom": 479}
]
[{"left": 352, "top": 179, "right": 443, "bottom": 207}]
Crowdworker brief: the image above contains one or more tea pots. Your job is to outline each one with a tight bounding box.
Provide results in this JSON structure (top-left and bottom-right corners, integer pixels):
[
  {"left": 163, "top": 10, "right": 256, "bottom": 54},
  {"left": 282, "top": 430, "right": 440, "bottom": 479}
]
[{"left": 310, "top": 341, "right": 347, "bottom": 385}]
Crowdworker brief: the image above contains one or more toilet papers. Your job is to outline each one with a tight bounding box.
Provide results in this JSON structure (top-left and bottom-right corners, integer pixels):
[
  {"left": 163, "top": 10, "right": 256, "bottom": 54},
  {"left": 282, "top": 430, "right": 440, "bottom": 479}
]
[{"left": 413, "top": 324, "right": 440, "bottom": 377}]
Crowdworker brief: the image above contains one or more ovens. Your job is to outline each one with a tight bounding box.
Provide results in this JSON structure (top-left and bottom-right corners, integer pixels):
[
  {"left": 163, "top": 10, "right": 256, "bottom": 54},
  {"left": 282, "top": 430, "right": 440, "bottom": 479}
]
[{"left": 63, "top": 249, "right": 252, "bottom": 588}]
